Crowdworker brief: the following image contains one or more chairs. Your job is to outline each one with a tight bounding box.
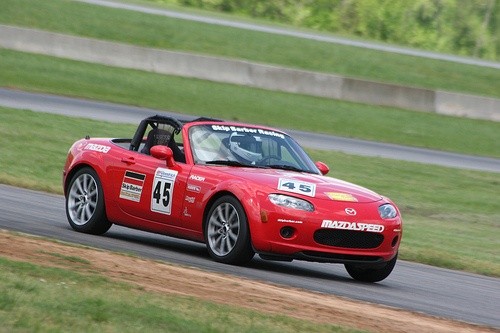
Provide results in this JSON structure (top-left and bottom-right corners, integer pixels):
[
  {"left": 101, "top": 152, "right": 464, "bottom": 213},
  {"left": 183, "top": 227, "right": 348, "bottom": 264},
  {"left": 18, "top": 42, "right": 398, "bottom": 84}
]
[
  {"left": 218, "top": 136, "right": 264, "bottom": 165},
  {"left": 141, "top": 128, "right": 185, "bottom": 164}
]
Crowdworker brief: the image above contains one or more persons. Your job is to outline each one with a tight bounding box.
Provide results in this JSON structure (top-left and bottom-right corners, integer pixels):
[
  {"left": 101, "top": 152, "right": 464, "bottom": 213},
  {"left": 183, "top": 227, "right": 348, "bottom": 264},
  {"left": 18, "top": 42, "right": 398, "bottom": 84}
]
[{"left": 223, "top": 129, "right": 267, "bottom": 165}]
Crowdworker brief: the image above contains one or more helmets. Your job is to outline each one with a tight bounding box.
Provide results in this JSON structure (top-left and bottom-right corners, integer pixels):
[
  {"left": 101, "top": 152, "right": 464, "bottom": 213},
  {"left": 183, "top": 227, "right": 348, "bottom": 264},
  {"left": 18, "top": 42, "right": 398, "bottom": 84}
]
[{"left": 228, "top": 131, "right": 263, "bottom": 162}]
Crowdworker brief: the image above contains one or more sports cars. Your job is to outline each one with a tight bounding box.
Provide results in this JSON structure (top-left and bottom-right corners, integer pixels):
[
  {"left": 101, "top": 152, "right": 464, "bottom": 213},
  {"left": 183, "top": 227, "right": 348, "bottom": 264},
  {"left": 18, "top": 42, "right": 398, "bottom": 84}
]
[{"left": 65, "top": 115, "right": 402, "bottom": 283}]
